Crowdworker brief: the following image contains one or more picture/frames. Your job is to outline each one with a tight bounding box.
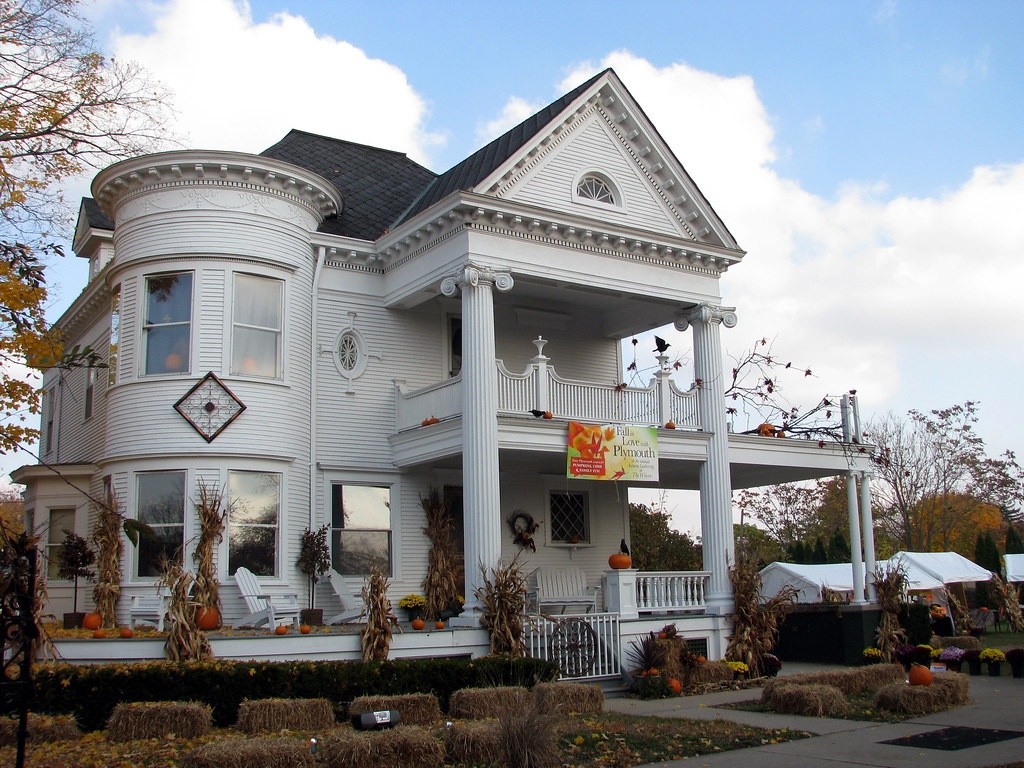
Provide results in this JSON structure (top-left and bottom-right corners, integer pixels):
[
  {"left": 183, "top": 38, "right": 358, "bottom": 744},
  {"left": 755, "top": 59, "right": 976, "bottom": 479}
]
[{"left": 950, "top": 660, "right": 962, "bottom": 673}]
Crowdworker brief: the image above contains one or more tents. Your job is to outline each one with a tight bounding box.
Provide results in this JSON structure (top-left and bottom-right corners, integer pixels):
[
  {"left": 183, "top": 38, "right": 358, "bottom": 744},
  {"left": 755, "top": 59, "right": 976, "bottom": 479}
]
[{"left": 751, "top": 551, "right": 1024, "bottom": 636}]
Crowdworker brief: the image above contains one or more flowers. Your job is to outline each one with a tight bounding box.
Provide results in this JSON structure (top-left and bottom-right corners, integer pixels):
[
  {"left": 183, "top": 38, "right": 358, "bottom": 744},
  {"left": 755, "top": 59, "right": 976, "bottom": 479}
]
[
  {"left": 979, "top": 648, "right": 1005, "bottom": 662},
  {"left": 399, "top": 594, "right": 426, "bottom": 608},
  {"left": 917, "top": 644, "right": 934, "bottom": 654},
  {"left": 894, "top": 645, "right": 915, "bottom": 657},
  {"left": 1006, "top": 649, "right": 1024, "bottom": 662},
  {"left": 446, "top": 595, "right": 465, "bottom": 613},
  {"left": 863, "top": 645, "right": 881, "bottom": 657},
  {"left": 726, "top": 661, "right": 749, "bottom": 673},
  {"left": 960, "top": 650, "right": 981, "bottom": 662},
  {"left": 939, "top": 647, "right": 966, "bottom": 660},
  {"left": 930, "top": 648, "right": 943, "bottom": 657}
]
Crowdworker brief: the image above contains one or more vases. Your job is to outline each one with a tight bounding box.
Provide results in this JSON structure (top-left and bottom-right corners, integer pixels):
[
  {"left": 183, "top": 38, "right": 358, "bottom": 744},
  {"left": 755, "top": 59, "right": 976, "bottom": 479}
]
[
  {"left": 870, "top": 657, "right": 880, "bottom": 664},
  {"left": 407, "top": 608, "right": 419, "bottom": 621},
  {"left": 1011, "top": 662, "right": 1024, "bottom": 678},
  {"left": 736, "top": 672, "right": 745, "bottom": 681},
  {"left": 969, "top": 658, "right": 981, "bottom": 675},
  {"left": 987, "top": 661, "right": 1001, "bottom": 676}
]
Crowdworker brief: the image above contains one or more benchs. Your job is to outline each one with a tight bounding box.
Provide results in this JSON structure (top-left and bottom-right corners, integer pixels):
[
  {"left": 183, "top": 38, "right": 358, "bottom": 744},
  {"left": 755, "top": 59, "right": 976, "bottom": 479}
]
[{"left": 532, "top": 565, "right": 602, "bottom": 614}]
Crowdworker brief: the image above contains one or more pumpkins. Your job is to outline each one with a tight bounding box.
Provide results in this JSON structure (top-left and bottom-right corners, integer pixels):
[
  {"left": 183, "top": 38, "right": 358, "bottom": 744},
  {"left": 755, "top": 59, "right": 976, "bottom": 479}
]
[
  {"left": 609, "top": 549, "right": 631, "bottom": 569},
  {"left": 423, "top": 418, "right": 429, "bottom": 426},
  {"left": 197, "top": 607, "right": 218, "bottom": 630},
  {"left": 668, "top": 678, "right": 680, "bottom": 691},
  {"left": 759, "top": 424, "right": 774, "bottom": 436},
  {"left": 666, "top": 422, "right": 674, "bottom": 428},
  {"left": 121, "top": 629, "right": 133, "bottom": 637},
  {"left": 543, "top": 412, "right": 552, "bottom": 419},
  {"left": 276, "top": 626, "right": 286, "bottom": 634},
  {"left": 412, "top": 616, "right": 424, "bottom": 630},
  {"left": 436, "top": 619, "right": 444, "bottom": 628},
  {"left": 778, "top": 432, "right": 785, "bottom": 438},
  {"left": 301, "top": 626, "right": 310, "bottom": 633},
  {"left": 94, "top": 630, "right": 105, "bottom": 638},
  {"left": 659, "top": 633, "right": 666, "bottom": 639},
  {"left": 909, "top": 662, "right": 932, "bottom": 685},
  {"left": 429, "top": 416, "right": 438, "bottom": 423},
  {"left": 84, "top": 612, "right": 101, "bottom": 629},
  {"left": 649, "top": 668, "right": 657, "bottom": 676},
  {"left": 240, "top": 358, "right": 256, "bottom": 373},
  {"left": 166, "top": 355, "right": 181, "bottom": 369},
  {"left": 697, "top": 656, "right": 705, "bottom": 663}
]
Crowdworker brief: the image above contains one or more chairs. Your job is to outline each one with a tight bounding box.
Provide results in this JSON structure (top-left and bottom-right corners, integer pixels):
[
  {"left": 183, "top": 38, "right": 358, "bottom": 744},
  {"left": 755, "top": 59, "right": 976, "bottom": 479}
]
[
  {"left": 231, "top": 566, "right": 302, "bottom": 634},
  {"left": 326, "top": 568, "right": 372, "bottom": 626},
  {"left": 128, "top": 569, "right": 198, "bottom": 633}
]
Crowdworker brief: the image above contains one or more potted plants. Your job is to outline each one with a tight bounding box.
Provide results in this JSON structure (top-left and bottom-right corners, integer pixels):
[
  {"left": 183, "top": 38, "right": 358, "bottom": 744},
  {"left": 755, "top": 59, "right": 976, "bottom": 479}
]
[
  {"left": 624, "top": 632, "right": 668, "bottom": 698},
  {"left": 56, "top": 528, "right": 99, "bottom": 629},
  {"left": 295, "top": 523, "right": 332, "bottom": 626}
]
